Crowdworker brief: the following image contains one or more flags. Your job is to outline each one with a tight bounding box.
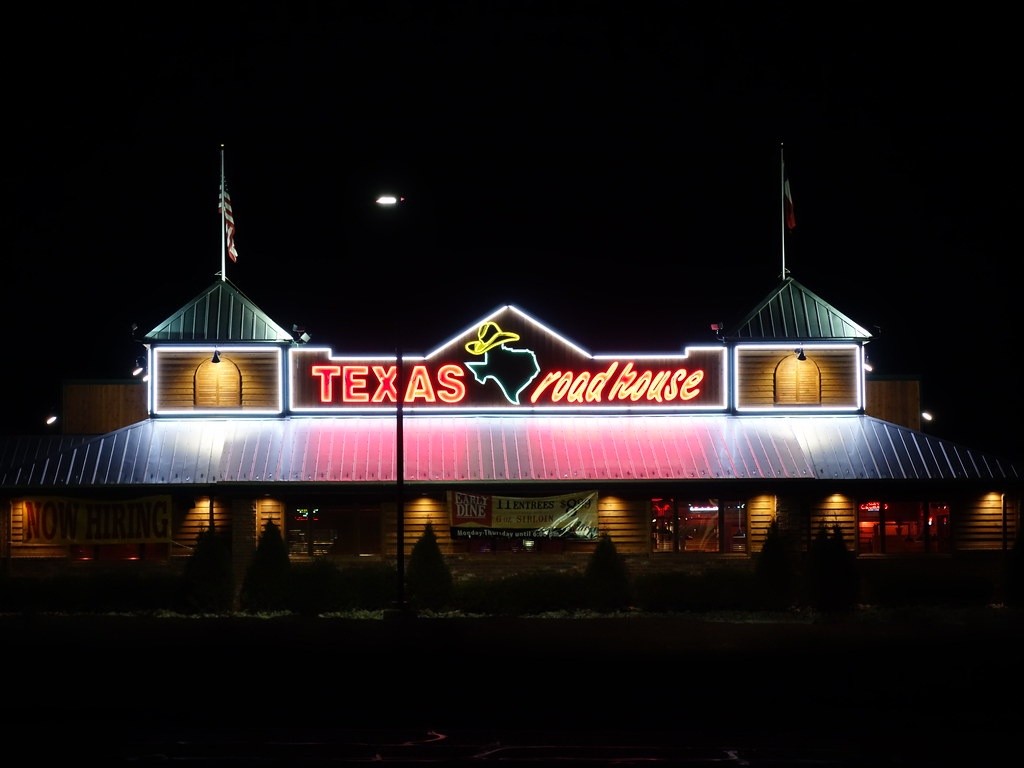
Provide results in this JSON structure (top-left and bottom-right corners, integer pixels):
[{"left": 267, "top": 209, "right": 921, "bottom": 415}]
[
  {"left": 218, "top": 164, "right": 238, "bottom": 262},
  {"left": 784, "top": 161, "right": 798, "bottom": 229}
]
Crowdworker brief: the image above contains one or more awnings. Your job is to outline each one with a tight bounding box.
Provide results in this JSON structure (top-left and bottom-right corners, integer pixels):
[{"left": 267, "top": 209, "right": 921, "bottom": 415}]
[{"left": 0, "top": 415, "right": 1024, "bottom": 490}]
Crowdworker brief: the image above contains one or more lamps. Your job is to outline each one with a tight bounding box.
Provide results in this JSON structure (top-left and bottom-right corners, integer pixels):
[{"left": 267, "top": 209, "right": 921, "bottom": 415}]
[
  {"left": 293, "top": 323, "right": 304, "bottom": 340},
  {"left": 129, "top": 323, "right": 137, "bottom": 331},
  {"left": 211, "top": 350, "right": 220, "bottom": 363},
  {"left": 133, "top": 354, "right": 148, "bottom": 383},
  {"left": 795, "top": 348, "right": 807, "bottom": 362}
]
